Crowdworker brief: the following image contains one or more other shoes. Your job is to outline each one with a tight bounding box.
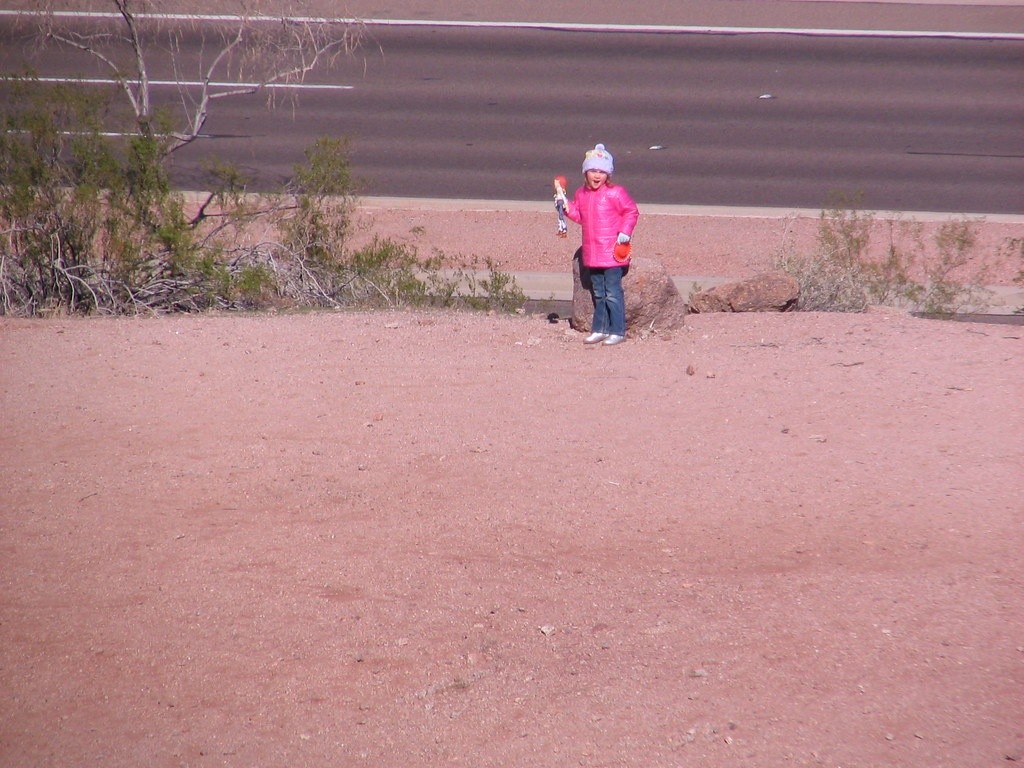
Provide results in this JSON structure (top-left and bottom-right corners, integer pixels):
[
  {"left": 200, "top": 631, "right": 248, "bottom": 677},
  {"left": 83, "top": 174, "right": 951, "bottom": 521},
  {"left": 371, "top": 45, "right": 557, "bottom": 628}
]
[
  {"left": 602, "top": 334, "right": 626, "bottom": 345},
  {"left": 583, "top": 332, "right": 609, "bottom": 344}
]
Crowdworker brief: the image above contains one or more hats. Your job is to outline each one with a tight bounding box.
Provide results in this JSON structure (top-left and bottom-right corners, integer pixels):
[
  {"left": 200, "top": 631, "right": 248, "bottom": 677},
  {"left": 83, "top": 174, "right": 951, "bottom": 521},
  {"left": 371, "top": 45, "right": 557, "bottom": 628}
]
[{"left": 582, "top": 144, "right": 614, "bottom": 177}]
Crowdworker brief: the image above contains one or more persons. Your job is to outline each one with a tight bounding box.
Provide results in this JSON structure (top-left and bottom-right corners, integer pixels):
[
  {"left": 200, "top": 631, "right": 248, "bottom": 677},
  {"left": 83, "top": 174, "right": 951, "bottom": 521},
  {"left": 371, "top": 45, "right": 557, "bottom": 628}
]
[
  {"left": 553, "top": 176, "right": 568, "bottom": 238},
  {"left": 552, "top": 144, "right": 640, "bottom": 345}
]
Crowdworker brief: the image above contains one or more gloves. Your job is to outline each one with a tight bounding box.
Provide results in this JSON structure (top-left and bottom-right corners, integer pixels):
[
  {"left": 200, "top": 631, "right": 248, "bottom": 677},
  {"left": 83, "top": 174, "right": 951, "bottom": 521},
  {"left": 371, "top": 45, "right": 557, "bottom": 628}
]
[
  {"left": 553, "top": 194, "right": 566, "bottom": 211},
  {"left": 618, "top": 232, "right": 630, "bottom": 245}
]
